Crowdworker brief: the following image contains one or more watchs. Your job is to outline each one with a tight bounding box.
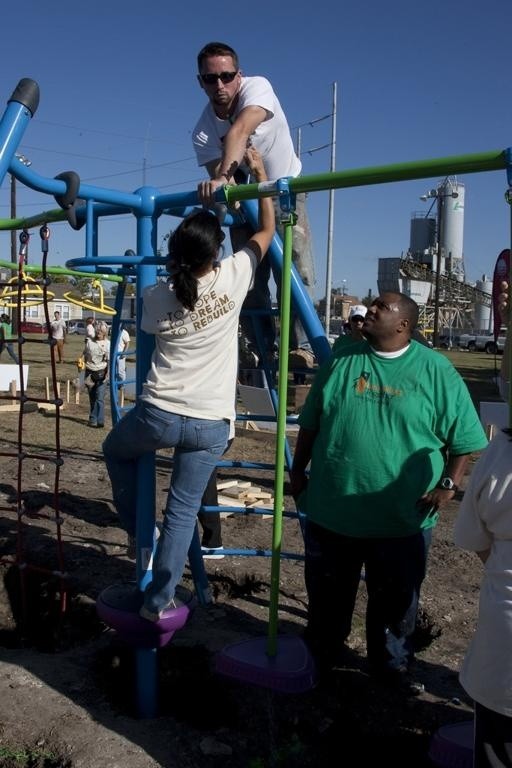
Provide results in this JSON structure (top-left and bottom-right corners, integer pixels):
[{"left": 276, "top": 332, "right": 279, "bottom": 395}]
[{"left": 437, "top": 477, "right": 458, "bottom": 494}]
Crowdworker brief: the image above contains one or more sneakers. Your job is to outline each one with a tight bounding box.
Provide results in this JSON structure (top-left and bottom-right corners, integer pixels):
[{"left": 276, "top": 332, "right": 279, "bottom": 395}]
[
  {"left": 140, "top": 581, "right": 177, "bottom": 623},
  {"left": 200, "top": 545, "right": 225, "bottom": 560},
  {"left": 128, "top": 527, "right": 161, "bottom": 559},
  {"left": 371, "top": 663, "right": 425, "bottom": 701}
]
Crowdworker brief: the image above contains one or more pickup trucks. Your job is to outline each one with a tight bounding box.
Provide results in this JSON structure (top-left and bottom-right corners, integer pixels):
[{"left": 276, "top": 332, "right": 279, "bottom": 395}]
[{"left": 475, "top": 326, "right": 507, "bottom": 354}]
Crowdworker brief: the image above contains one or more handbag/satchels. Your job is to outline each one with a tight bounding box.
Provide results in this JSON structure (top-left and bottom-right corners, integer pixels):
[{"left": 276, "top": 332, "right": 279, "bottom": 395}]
[{"left": 85, "top": 368, "right": 107, "bottom": 391}]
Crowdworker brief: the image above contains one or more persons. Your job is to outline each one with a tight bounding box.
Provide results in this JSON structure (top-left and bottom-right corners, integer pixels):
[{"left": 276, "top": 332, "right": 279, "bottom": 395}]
[
  {"left": 498, "top": 275, "right": 512, "bottom": 411},
  {"left": 116, "top": 326, "right": 131, "bottom": 407},
  {"left": 331, "top": 304, "right": 370, "bottom": 348},
  {"left": 102, "top": 146, "right": 276, "bottom": 621},
  {"left": 293, "top": 289, "right": 494, "bottom": 710},
  {"left": 84, "top": 316, "right": 95, "bottom": 336},
  {"left": 342, "top": 323, "right": 352, "bottom": 335},
  {"left": 454, "top": 425, "right": 510, "bottom": 768},
  {"left": 76, "top": 320, "right": 109, "bottom": 428},
  {"left": 48, "top": 311, "right": 66, "bottom": 363},
  {"left": 190, "top": 41, "right": 315, "bottom": 371},
  {"left": 0, "top": 314, "right": 20, "bottom": 366},
  {"left": 75, "top": 324, "right": 94, "bottom": 404}
]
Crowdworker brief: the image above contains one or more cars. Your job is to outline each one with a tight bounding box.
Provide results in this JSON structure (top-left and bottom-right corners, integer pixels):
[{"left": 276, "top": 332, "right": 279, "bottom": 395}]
[
  {"left": 439, "top": 335, "right": 449, "bottom": 346},
  {"left": 457, "top": 330, "right": 492, "bottom": 352},
  {"left": 104, "top": 319, "right": 112, "bottom": 336},
  {"left": 427, "top": 339, "right": 433, "bottom": 349},
  {"left": 21, "top": 320, "right": 47, "bottom": 334},
  {"left": 66, "top": 320, "right": 87, "bottom": 335}
]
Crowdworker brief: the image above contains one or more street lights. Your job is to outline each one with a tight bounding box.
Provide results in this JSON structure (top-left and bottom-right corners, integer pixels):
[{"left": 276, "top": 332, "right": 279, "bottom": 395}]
[
  {"left": 341, "top": 279, "right": 347, "bottom": 294},
  {"left": 420, "top": 185, "right": 462, "bottom": 349}
]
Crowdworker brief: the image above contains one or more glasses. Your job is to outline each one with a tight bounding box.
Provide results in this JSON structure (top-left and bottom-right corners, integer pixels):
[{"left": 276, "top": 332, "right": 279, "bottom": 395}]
[{"left": 199, "top": 72, "right": 239, "bottom": 84}]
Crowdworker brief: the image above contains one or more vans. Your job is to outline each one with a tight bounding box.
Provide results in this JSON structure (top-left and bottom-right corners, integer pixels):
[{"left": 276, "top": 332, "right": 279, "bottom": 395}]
[{"left": 122, "top": 319, "right": 136, "bottom": 337}]
